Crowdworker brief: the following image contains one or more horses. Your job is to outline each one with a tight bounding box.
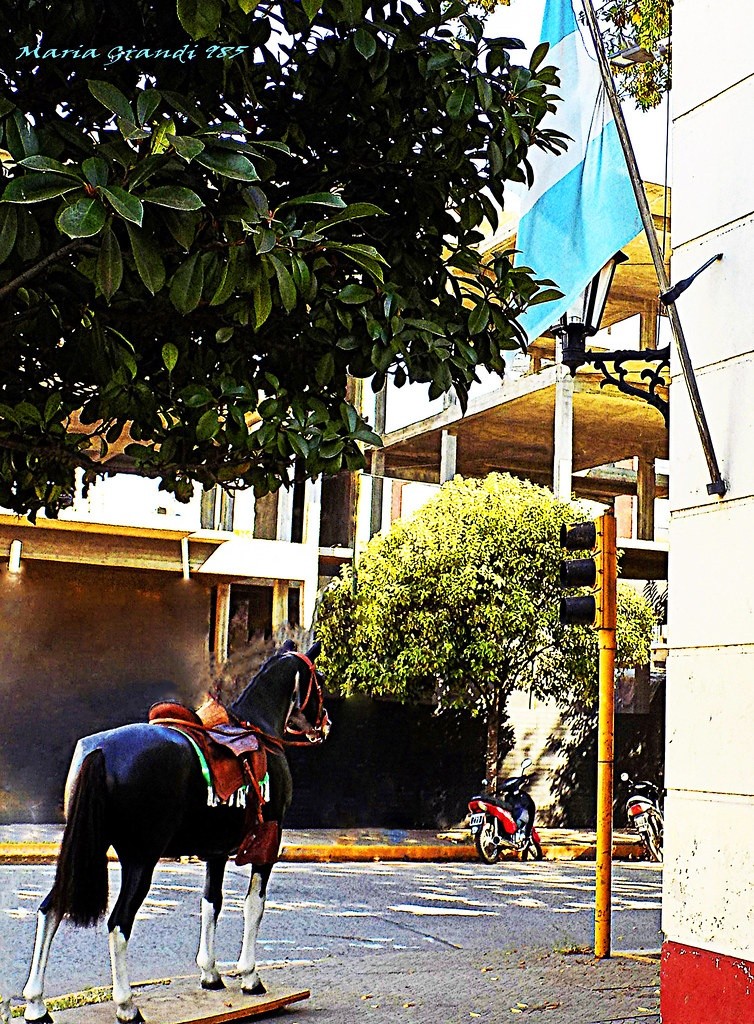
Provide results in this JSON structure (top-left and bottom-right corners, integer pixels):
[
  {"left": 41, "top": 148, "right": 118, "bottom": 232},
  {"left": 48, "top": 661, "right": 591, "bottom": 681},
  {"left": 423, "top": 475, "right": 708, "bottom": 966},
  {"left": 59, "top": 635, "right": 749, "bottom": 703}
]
[{"left": 23, "top": 639, "right": 333, "bottom": 1024}]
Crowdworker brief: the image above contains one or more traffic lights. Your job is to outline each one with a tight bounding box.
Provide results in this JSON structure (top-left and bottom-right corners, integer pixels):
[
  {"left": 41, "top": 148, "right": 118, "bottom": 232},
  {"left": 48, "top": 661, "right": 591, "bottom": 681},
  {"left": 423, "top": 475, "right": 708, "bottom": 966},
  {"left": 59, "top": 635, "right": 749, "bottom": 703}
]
[{"left": 559, "top": 517, "right": 602, "bottom": 629}]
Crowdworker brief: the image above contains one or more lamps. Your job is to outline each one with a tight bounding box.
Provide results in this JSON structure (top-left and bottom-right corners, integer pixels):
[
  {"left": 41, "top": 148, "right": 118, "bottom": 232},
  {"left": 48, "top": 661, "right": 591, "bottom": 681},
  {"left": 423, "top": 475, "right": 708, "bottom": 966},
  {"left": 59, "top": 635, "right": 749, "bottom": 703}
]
[{"left": 549, "top": 249, "right": 630, "bottom": 378}]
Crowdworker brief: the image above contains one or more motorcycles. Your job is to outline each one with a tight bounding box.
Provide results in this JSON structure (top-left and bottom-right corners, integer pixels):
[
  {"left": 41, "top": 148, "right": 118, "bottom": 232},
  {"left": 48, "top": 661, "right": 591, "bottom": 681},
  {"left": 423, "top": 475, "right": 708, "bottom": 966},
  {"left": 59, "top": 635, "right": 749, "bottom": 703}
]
[
  {"left": 468, "top": 758, "right": 543, "bottom": 865},
  {"left": 620, "top": 772, "right": 663, "bottom": 864}
]
